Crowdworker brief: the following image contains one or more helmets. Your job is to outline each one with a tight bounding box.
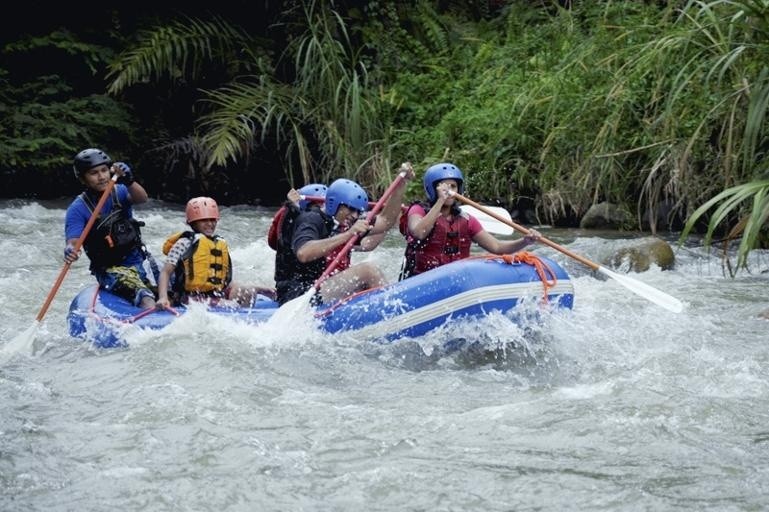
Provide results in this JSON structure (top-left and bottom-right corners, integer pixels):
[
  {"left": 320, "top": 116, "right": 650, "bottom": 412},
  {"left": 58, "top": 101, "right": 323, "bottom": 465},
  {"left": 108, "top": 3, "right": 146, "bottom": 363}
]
[
  {"left": 422, "top": 161, "right": 464, "bottom": 204},
  {"left": 296, "top": 182, "right": 329, "bottom": 211},
  {"left": 185, "top": 196, "right": 222, "bottom": 224},
  {"left": 325, "top": 178, "right": 369, "bottom": 217},
  {"left": 72, "top": 148, "right": 114, "bottom": 179}
]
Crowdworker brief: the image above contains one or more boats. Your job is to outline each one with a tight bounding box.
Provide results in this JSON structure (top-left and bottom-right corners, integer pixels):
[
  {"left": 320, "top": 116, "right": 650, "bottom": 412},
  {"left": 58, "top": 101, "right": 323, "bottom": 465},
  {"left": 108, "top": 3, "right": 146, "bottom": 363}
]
[{"left": 65, "top": 251, "right": 579, "bottom": 365}]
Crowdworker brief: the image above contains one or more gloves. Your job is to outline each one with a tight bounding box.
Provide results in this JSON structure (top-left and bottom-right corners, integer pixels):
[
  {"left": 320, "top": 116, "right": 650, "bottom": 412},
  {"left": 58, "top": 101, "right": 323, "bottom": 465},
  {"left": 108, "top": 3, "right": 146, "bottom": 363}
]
[
  {"left": 115, "top": 161, "right": 135, "bottom": 188},
  {"left": 64, "top": 244, "right": 82, "bottom": 256}
]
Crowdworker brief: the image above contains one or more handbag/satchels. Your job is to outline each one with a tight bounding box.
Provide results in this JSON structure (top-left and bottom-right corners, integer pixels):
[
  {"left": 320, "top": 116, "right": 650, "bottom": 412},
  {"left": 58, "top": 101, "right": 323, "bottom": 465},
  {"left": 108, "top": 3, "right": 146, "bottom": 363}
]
[{"left": 94, "top": 207, "right": 143, "bottom": 269}]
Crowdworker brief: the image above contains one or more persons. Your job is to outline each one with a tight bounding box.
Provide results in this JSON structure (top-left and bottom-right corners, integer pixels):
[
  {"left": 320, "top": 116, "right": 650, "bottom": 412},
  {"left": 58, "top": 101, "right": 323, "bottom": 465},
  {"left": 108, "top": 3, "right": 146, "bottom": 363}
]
[
  {"left": 59, "top": 145, "right": 161, "bottom": 312},
  {"left": 399, "top": 163, "right": 541, "bottom": 282},
  {"left": 293, "top": 181, "right": 388, "bottom": 275},
  {"left": 152, "top": 196, "right": 277, "bottom": 312},
  {"left": 274, "top": 164, "right": 416, "bottom": 310}
]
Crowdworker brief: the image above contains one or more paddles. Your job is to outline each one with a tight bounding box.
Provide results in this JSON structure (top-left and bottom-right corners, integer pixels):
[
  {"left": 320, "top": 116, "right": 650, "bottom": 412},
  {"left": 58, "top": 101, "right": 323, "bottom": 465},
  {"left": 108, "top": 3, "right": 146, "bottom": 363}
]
[
  {"left": 287, "top": 187, "right": 514, "bottom": 236},
  {"left": 262, "top": 163, "right": 413, "bottom": 332},
  {"left": 448, "top": 192, "right": 685, "bottom": 315},
  {"left": 8, "top": 172, "right": 120, "bottom": 353}
]
